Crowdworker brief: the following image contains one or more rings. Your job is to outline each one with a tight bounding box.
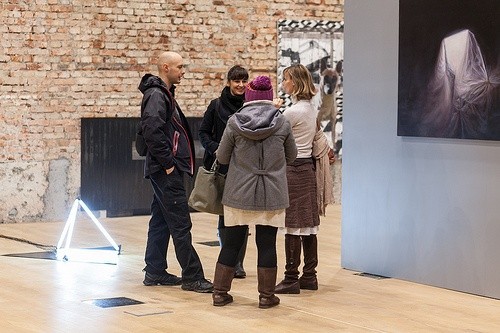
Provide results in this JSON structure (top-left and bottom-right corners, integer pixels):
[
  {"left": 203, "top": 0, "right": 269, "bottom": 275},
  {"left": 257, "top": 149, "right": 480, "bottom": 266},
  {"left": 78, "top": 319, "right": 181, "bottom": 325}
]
[{"left": 278, "top": 100, "right": 280, "bottom": 102}]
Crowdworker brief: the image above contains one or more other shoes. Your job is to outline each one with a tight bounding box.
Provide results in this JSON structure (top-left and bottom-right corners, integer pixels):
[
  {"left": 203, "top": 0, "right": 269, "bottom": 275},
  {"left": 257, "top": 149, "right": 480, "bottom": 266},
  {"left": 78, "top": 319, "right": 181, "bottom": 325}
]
[{"left": 234, "top": 265, "right": 247, "bottom": 277}]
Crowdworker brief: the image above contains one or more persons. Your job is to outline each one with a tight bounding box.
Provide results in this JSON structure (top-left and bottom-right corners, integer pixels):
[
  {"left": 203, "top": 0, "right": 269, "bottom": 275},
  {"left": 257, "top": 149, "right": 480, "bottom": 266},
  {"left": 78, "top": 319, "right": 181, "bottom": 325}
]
[
  {"left": 138, "top": 51, "right": 214, "bottom": 292},
  {"left": 200, "top": 64, "right": 249, "bottom": 278},
  {"left": 273, "top": 63, "right": 320, "bottom": 294},
  {"left": 212, "top": 76, "right": 297, "bottom": 309}
]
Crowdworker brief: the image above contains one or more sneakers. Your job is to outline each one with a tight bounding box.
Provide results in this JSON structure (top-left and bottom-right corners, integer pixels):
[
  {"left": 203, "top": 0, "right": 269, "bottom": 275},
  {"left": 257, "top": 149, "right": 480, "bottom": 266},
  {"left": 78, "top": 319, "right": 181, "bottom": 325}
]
[
  {"left": 143, "top": 269, "right": 182, "bottom": 286},
  {"left": 181, "top": 278, "right": 214, "bottom": 292}
]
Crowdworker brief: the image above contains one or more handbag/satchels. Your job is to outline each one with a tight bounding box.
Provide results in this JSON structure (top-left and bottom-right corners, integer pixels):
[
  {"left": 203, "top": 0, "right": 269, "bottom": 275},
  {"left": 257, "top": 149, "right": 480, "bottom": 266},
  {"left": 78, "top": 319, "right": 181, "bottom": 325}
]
[
  {"left": 135, "top": 132, "right": 148, "bottom": 156},
  {"left": 190, "top": 158, "right": 225, "bottom": 215}
]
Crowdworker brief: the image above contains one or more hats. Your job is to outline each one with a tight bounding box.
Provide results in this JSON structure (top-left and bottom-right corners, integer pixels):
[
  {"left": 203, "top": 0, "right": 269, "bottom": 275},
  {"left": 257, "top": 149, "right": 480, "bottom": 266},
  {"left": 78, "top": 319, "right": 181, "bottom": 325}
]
[{"left": 244, "top": 75, "right": 273, "bottom": 102}]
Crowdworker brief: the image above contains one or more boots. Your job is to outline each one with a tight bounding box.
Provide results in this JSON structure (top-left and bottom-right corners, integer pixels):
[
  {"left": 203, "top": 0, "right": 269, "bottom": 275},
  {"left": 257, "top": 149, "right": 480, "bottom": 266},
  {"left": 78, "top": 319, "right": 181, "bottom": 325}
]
[
  {"left": 257, "top": 266, "right": 280, "bottom": 308},
  {"left": 213, "top": 263, "right": 234, "bottom": 306},
  {"left": 299, "top": 235, "right": 318, "bottom": 289},
  {"left": 274, "top": 236, "right": 301, "bottom": 294}
]
